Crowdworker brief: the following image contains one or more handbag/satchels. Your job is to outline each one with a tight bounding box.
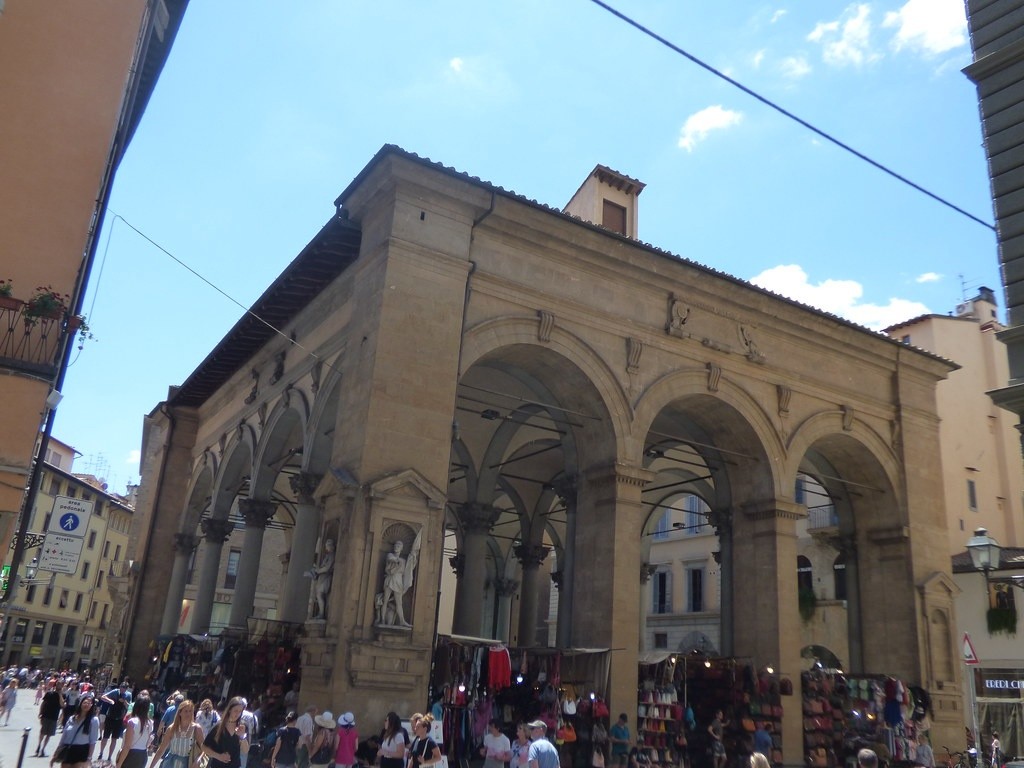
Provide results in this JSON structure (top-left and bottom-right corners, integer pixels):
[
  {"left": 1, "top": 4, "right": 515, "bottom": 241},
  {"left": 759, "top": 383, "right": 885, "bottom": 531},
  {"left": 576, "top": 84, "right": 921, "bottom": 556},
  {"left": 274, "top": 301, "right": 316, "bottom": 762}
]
[
  {"left": 1, "top": 700, "right": 7, "bottom": 706},
  {"left": 311, "top": 745, "right": 330, "bottom": 764},
  {"left": 194, "top": 750, "right": 212, "bottom": 768},
  {"left": 116, "top": 749, "right": 123, "bottom": 764},
  {"left": 637, "top": 682, "right": 695, "bottom": 768},
  {"left": 54, "top": 747, "right": 70, "bottom": 763},
  {"left": 539, "top": 680, "right": 608, "bottom": 768},
  {"left": 418, "top": 755, "right": 448, "bottom": 768}
]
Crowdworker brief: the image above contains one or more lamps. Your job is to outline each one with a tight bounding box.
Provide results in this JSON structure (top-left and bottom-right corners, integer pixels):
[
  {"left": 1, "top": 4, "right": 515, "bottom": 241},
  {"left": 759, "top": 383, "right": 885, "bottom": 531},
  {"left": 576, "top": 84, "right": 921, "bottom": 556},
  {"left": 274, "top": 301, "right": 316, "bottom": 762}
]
[
  {"left": 648, "top": 450, "right": 664, "bottom": 459},
  {"left": 482, "top": 410, "right": 499, "bottom": 419},
  {"left": 672, "top": 523, "right": 684, "bottom": 529},
  {"left": 47, "top": 389, "right": 63, "bottom": 410},
  {"left": 542, "top": 484, "right": 554, "bottom": 491},
  {"left": 962, "top": 525, "right": 1024, "bottom": 598}
]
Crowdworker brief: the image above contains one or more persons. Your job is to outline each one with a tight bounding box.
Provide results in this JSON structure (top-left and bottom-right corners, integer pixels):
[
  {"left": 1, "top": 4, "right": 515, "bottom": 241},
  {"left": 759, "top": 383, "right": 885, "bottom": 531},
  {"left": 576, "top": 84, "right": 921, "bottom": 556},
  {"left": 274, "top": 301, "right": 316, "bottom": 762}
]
[
  {"left": 916, "top": 734, "right": 935, "bottom": 768},
  {"left": 991, "top": 732, "right": 1000, "bottom": 768},
  {"left": 629, "top": 739, "right": 659, "bottom": 768},
  {"left": 708, "top": 709, "right": 730, "bottom": 768},
  {"left": 858, "top": 749, "right": 878, "bottom": 768},
  {"left": 966, "top": 728, "right": 973, "bottom": 749},
  {"left": 308, "top": 539, "right": 335, "bottom": 618},
  {"left": 381, "top": 541, "right": 414, "bottom": 627},
  {"left": 0, "top": 665, "right": 441, "bottom": 768},
  {"left": 870, "top": 734, "right": 889, "bottom": 768},
  {"left": 480, "top": 719, "right": 561, "bottom": 768},
  {"left": 750, "top": 752, "right": 770, "bottom": 768},
  {"left": 755, "top": 722, "right": 773, "bottom": 762},
  {"left": 609, "top": 713, "right": 632, "bottom": 768}
]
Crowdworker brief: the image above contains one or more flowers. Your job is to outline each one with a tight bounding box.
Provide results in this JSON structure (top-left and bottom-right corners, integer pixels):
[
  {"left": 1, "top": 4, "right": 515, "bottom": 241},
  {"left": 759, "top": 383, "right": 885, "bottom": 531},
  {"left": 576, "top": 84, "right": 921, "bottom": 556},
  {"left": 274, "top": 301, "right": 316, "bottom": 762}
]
[
  {"left": 0, "top": 278, "right": 14, "bottom": 297},
  {"left": 62, "top": 313, "right": 96, "bottom": 347},
  {"left": 25, "top": 283, "right": 67, "bottom": 326}
]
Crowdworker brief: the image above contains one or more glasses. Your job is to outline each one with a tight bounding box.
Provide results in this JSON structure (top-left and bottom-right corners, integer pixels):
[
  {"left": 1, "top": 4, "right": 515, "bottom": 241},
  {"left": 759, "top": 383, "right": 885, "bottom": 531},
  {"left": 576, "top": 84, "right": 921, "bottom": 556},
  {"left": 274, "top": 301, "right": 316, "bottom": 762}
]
[{"left": 528, "top": 727, "right": 537, "bottom": 732}]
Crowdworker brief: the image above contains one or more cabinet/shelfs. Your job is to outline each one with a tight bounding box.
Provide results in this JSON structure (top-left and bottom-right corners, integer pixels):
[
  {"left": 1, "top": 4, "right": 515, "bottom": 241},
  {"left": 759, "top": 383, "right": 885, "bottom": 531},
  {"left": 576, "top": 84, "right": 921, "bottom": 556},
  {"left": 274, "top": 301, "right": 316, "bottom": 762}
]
[{"left": 639, "top": 685, "right": 687, "bottom": 768}]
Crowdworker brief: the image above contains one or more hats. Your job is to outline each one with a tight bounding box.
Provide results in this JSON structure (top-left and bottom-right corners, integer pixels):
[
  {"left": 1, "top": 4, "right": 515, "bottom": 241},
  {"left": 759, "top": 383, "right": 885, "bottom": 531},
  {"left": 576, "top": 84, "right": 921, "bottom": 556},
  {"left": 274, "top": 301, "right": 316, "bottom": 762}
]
[
  {"left": 315, "top": 711, "right": 336, "bottom": 729},
  {"left": 620, "top": 713, "right": 628, "bottom": 722},
  {"left": 527, "top": 720, "right": 547, "bottom": 733},
  {"left": 337, "top": 712, "right": 356, "bottom": 736}
]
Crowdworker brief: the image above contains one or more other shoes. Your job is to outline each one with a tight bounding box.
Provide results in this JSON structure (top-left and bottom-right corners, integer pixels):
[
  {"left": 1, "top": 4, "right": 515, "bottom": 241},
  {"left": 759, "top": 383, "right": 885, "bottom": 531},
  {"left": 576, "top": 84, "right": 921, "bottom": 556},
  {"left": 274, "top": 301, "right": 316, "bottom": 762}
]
[
  {"left": 36, "top": 748, "right": 40, "bottom": 756},
  {"left": 107, "top": 758, "right": 111, "bottom": 761},
  {"left": 39, "top": 749, "right": 48, "bottom": 757},
  {"left": 98, "top": 737, "right": 102, "bottom": 740},
  {"left": 97, "top": 755, "right": 102, "bottom": 760}
]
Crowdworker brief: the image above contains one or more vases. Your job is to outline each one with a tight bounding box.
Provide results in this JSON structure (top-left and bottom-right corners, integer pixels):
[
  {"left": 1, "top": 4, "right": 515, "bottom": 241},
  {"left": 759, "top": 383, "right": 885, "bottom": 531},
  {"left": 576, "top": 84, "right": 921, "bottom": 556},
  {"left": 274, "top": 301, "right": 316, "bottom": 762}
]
[
  {"left": 30, "top": 305, "right": 61, "bottom": 318},
  {"left": 0, "top": 295, "right": 23, "bottom": 311},
  {"left": 65, "top": 317, "right": 82, "bottom": 329}
]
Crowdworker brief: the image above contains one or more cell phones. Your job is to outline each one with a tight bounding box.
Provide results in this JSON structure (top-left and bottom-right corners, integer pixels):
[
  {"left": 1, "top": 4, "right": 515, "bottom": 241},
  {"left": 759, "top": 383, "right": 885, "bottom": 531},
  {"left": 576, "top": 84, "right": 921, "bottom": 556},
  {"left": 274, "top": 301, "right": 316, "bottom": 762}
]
[{"left": 239, "top": 725, "right": 244, "bottom": 731}]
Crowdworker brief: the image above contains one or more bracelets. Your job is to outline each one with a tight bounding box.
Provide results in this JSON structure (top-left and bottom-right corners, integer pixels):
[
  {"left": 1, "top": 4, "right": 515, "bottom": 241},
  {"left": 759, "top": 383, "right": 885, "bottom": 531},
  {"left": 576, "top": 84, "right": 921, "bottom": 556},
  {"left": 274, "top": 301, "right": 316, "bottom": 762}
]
[
  {"left": 240, "top": 737, "right": 246, "bottom": 740},
  {"left": 88, "top": 757, "right": 92, "bottom": 760}
]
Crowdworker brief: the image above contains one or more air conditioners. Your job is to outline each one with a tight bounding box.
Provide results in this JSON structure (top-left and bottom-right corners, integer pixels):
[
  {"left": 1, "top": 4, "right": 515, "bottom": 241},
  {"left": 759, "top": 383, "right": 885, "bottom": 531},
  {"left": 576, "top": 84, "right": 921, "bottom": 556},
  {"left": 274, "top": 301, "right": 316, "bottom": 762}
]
[{"left": 955, "top": 300, "right": 976, "bottom": 317}]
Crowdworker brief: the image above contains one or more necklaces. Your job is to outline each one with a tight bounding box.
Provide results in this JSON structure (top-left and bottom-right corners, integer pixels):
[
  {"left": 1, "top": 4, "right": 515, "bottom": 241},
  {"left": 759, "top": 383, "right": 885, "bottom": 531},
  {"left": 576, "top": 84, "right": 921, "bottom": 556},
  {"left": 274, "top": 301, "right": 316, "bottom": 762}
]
[
  {"left": 179, "top": 724, "right": 190, "bottom": 738},
  {"left": 229, "top": 721, "right": 236, "bottom": 723}
]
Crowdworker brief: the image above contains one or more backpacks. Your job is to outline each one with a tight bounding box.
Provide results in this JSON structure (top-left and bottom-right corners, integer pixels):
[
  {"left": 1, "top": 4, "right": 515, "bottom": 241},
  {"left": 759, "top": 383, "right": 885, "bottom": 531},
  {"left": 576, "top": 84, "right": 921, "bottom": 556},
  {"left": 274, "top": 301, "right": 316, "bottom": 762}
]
[{"left": 107, "top": 694, "right": 127, "bottom": 720}]
